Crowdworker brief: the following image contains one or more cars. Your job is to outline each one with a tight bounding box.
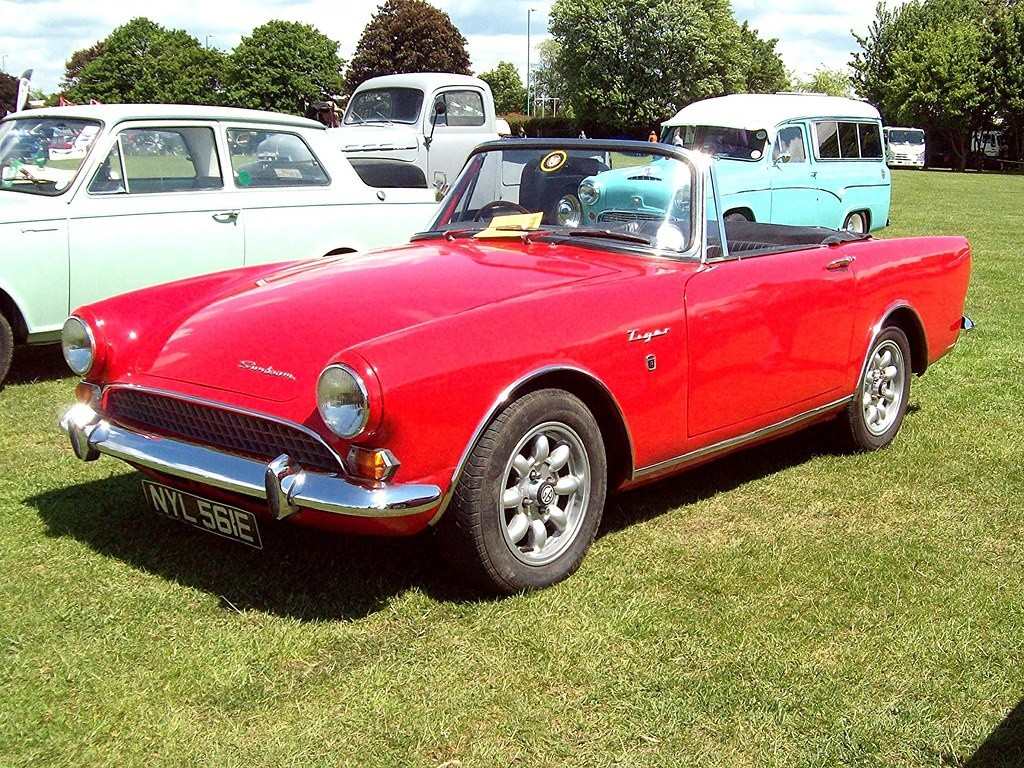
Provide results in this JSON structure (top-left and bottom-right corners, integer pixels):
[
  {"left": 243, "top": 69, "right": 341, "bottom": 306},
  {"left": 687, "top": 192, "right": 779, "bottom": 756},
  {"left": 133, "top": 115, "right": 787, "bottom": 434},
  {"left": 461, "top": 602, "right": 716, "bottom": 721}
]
[
  {"left": 347, "top": 74, "right": 512, "bottom": 192},
  {"left": 0, "top": 104, "right": 446, "bottom": 388},
  {"left": 575, "top": 92, "right": 894, "bottom": 251},
  {"left": 59, "top": 136, "right": 974, "bottom": 588}
]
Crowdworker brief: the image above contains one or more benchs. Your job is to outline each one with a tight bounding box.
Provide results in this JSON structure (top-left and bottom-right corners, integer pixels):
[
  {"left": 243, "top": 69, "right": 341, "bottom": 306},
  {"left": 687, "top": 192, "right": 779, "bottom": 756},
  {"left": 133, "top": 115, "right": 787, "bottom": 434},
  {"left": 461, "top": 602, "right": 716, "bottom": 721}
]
[{"left": 613, "top": 218, "right": 834, "bottom": 263}]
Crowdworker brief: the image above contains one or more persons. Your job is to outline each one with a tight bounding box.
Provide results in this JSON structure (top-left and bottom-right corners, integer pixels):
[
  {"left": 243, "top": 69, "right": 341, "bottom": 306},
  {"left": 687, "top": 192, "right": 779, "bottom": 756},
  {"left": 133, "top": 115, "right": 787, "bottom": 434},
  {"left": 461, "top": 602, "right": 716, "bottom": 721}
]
[{"left": 648, "top": 131, "right": 683, "bottom": 159}]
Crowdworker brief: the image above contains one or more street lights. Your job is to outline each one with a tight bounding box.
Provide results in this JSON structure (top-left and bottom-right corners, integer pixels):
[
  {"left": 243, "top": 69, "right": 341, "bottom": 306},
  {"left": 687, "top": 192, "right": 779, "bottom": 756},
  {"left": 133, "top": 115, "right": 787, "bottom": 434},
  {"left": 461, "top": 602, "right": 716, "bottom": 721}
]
[{"left": 524, "top": 6, "right": 539, "bottom": 121}]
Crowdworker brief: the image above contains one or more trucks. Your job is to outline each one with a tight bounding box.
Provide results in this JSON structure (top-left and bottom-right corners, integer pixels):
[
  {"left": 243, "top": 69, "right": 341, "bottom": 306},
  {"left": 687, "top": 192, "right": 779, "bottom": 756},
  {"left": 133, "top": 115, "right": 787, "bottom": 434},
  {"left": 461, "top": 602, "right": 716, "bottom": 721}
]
[{"left": 882, "top": 123, "right": 927, "bottom": 173}]
